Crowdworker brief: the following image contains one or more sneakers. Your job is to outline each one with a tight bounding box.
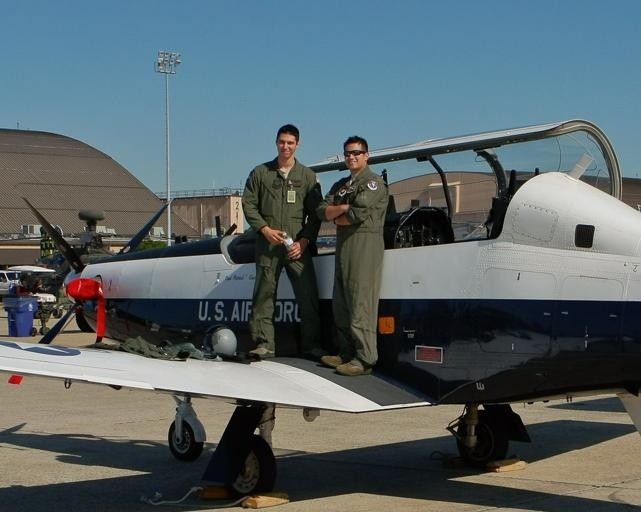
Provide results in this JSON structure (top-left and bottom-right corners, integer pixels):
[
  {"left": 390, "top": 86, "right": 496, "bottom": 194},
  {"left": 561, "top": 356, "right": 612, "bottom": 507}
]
[
  {"left": 320, "top": 351, "right": 373, "bottom": 375},
  {"left": 248, "top": 343, "right": 276, "bottom": 358}
]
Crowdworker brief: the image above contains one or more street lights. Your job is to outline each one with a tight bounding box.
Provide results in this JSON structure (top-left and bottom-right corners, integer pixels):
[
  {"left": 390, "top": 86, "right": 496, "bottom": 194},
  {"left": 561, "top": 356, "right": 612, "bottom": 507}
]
[{"left": 155, "top": 49, "right": 182, "bottom": 246}]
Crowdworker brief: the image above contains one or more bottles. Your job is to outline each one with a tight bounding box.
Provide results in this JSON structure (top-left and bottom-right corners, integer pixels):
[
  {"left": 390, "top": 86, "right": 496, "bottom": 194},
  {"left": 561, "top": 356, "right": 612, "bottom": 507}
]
[{"left": 283, "top": 232, "right": 301, "bottom": 260}]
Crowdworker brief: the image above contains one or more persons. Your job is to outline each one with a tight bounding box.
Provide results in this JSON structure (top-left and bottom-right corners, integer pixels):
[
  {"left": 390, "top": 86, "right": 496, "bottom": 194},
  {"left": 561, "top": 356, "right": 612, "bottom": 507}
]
[
  {"left": 240, "top": 124, "right": 330, "bottom": 360},
  {"left": 319, "top": 135, "right": 392, "bottom": 376}
]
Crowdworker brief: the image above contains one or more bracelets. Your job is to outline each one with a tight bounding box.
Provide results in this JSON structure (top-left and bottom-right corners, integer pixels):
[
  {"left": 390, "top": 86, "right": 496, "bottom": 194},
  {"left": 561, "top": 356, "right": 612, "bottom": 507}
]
[{"left": 333, "top": 219, "right": 337, "bottom": 224}]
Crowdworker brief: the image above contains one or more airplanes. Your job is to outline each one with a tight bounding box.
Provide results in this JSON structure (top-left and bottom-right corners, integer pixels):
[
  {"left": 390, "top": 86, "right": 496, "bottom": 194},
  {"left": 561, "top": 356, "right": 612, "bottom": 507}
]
[{"left": 0, "top": 120, "right": 641, "bottom": 500}]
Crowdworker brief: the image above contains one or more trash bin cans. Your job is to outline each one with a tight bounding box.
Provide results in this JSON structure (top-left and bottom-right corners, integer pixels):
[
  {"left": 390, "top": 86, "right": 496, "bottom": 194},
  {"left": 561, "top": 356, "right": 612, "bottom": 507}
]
[{"left": 2, "top": 296, "right": 39, "bottom": 337}]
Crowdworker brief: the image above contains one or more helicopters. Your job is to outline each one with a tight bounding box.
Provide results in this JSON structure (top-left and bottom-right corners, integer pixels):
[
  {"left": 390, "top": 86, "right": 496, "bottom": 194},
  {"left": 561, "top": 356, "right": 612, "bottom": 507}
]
[{"left": 1, "top": 208, "right": 136, "bottom": 319}]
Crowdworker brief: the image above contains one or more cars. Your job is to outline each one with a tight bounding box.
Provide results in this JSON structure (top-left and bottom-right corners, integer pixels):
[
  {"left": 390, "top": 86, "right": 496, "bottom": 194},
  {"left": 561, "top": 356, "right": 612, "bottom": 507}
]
[{"left": 0, "top": 269, "right": 22, "bottom": 295}]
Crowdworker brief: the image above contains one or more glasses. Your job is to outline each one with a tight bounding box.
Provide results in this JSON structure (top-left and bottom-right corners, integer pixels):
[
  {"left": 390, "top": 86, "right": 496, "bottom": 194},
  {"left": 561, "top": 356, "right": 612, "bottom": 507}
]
[{"left": 343, "top": 150, "right": 367, "bottom": 156}]
